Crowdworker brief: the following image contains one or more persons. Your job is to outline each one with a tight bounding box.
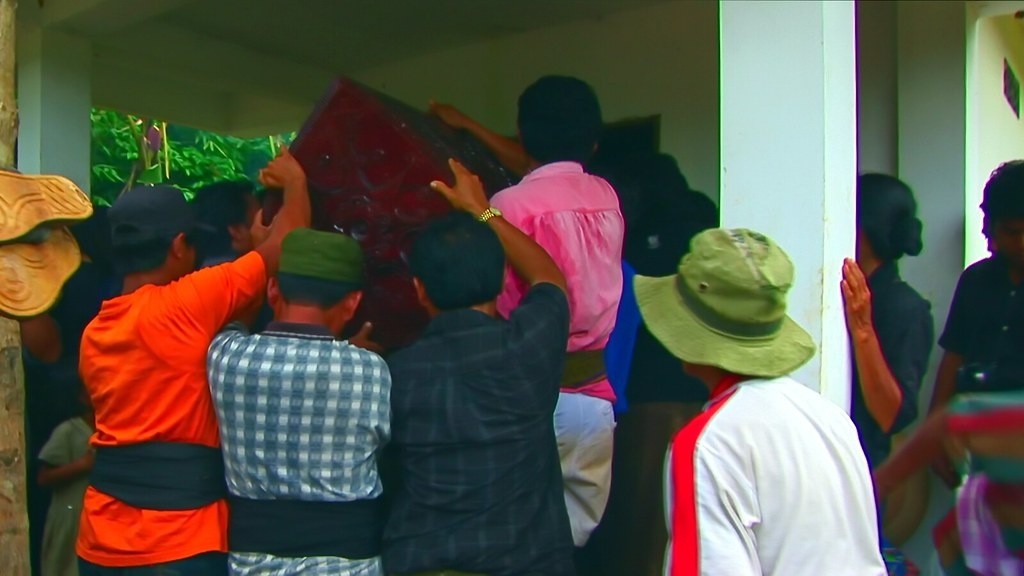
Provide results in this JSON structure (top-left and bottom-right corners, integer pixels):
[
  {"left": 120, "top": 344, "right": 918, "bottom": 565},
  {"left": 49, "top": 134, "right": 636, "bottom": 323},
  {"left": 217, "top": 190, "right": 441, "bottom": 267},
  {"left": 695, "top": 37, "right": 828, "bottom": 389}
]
[
  {"left": 840, "top": 159, "right": 1024, "bottom": 576},
  {"left": 632, "top": 230, "right": 891, "bottom": 576},
  {"left": 17, "top": 74, "right": 641, "bottom": 576}
]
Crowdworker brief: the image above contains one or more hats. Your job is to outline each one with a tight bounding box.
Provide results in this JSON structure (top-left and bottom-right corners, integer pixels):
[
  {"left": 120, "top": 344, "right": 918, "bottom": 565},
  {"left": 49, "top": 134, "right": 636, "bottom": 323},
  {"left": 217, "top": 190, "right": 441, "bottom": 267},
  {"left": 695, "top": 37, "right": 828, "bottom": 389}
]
[
  {"left": 108, "top": 184, "right": 217, "bottom": 240},
  {"left": 279, "top": 227, "right": 364, "bottom": 284},
  {"left": 634, "top": 229, "right": 814, "bottom": 377}
]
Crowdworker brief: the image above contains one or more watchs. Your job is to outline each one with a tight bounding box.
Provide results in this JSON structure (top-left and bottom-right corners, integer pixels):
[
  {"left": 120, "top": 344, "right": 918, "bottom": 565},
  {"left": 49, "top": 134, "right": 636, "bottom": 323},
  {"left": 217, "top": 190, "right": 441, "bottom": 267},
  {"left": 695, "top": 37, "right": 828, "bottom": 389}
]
[{"left": 478, "top": 206, "right": 503, "bottom": 223}]
